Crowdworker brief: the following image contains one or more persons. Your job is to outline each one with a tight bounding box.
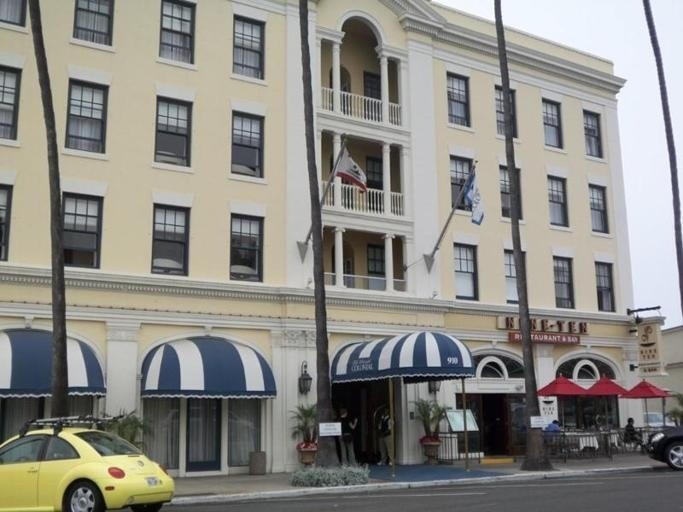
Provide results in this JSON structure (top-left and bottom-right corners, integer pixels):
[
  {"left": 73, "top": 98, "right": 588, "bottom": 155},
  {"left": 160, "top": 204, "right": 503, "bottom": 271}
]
[
  {"left": 334, "top": 408, "right": 359, "bottom": 466},
  {"left": 545, "top": 417, "right": 561, "bottom": 435},
  {"left": 376, "top": 407, "right": 396, "bottom": 467},
  {"left": 620, "top": 417, "right": 644, "bottom": 452}
]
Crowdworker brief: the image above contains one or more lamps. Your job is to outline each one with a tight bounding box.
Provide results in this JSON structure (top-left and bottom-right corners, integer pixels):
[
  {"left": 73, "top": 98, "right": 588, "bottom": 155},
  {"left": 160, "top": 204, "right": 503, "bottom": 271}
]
[{"left": 297, "top": 360, "right": 312, "bottom": 394}]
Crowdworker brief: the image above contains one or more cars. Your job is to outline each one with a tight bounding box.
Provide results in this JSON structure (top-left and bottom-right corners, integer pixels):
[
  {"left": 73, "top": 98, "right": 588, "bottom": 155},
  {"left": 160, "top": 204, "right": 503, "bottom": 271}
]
[
  {"left": 0, "top": 415, "right": 176, "bottom": 512},
  {"left": 644, "top": 411, "right": 683, "bottom": 471}
]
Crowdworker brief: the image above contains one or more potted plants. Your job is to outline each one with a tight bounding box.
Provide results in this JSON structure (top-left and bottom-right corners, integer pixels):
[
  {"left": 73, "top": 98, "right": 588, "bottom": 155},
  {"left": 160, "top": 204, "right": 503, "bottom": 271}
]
[
  {"left": 288, "top": 402, "right": 323, "bottom": 469},
  {"left": 408, "top": 398, "right": 452, "bottom": 464}
]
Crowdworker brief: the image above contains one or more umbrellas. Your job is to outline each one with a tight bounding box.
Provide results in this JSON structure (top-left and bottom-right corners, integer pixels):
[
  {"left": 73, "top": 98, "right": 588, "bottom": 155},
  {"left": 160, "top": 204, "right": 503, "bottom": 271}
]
[
  {"left": 537, "top": 375, "right": 590, "bottom": 427},
  {"left": 620, "top": 378, "right": 672, "bottom": 426},
  {"left": 584, "top": 377, "right": 627, "bottom": 429}
]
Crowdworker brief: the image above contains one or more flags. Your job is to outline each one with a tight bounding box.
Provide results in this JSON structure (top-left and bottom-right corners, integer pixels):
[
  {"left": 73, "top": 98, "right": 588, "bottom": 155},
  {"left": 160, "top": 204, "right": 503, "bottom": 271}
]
[
  {"left": 336, "top": 145, "right": 369, "bottom": 195},
  {"left": 462, "top": 166, "right": 486, "bottom": 227}
]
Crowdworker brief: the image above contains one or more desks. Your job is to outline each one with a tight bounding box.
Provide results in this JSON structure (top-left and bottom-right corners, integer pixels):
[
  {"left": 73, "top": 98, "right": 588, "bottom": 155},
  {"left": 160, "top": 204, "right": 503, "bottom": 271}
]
[{"left": 560, "top": 431, "right": 597, "bottom": 457}]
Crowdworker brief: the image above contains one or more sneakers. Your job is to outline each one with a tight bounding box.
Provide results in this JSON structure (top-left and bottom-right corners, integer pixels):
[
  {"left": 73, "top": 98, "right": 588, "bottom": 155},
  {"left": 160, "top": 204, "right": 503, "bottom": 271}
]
[{"left": 376, "top": 459, "right": 393, "bottom": 468}]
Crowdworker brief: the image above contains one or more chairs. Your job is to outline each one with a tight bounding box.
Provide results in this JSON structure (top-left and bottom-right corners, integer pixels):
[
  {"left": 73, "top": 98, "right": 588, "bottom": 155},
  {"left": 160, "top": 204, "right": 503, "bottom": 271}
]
[{"left": 616, "top": 430, "right": 636, "bottom": 452}]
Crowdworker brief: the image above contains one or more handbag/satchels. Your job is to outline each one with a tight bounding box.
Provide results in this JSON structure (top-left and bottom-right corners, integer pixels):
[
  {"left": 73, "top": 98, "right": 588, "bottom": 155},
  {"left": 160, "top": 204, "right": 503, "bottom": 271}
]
[{"left": 343, "top": 433, "right": 353, "bottom": 444}]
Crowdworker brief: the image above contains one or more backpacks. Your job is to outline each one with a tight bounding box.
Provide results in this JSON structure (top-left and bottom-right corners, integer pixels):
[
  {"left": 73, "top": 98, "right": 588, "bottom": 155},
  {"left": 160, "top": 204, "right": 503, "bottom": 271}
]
[{"left": 380, "top": 415, "right": 390, "bottom": 432}]
